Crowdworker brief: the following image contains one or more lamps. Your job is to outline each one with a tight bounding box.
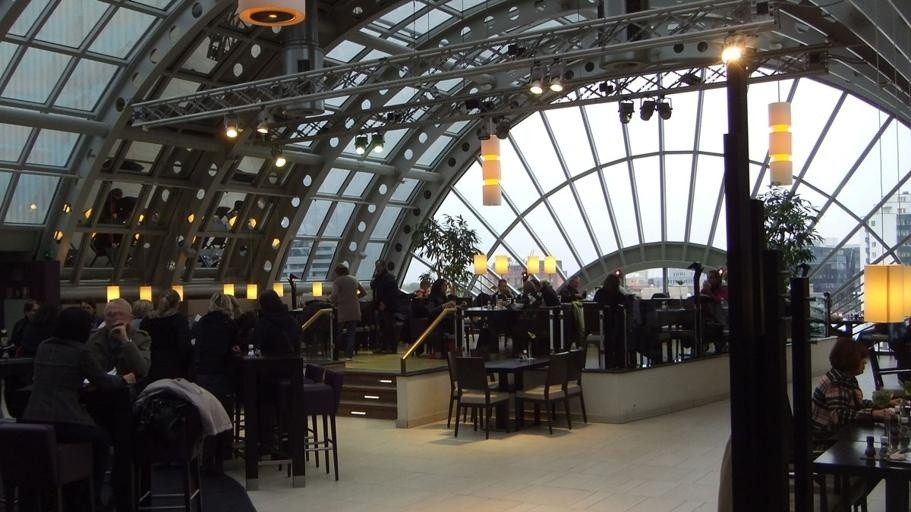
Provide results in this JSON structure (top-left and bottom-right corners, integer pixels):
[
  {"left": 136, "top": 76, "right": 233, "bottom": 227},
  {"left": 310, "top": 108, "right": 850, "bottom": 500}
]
[
  {"left": 549, "top": 59, "right": 566, "bottom": 92},
  {"left": 481, "top": 116, "right": 501, "bottom": 206},
  {"left": 237, "top": 0, "right": 307, "bottom": 28},
  {"left": 107, "top": 281, "right": 324, "bottom": 301},
  {"left": 863, "top": 0, "right": 905, "bottom": 323},
  {"left": 53, "top": 201, "right": 72, "bottom": 220},
  {"left": 472, "top": 252, "right": 556, "bottom": 293},
  {"left": 892, "top": 0, "right": 911, "bottom": 321},
  {"left": 618, "top": 98, "right": 674, "bottom": 124},
  {"left": 256, "top": 110, "right": 269, "bottom": 134},
  {"left": 223, "top": 114, "right": 240, "bottom": 139},
  {"left": 529, "top": 61, "right": 546, "bottom": 95},
  {"left": 271, "top": 144, "right": 287, "bottom": 168},
  {"left": 721, "top": 32, "right": 745, "bottom": 65},
  {"left": 768, "top": 42, "right": 793, "bottom": 185},
  {"left": 354, "top": 131, "right": 385, "bottom": 155},
  {"left": 49, "top": 225, "right": 64, "bottom": 243}
]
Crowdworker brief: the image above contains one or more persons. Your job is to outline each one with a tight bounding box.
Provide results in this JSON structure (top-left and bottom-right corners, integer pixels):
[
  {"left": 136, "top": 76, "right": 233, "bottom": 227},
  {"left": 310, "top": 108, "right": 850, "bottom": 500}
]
[
  {"left": 812, "top": 335, "right": 910, "bottom": 512},
  {"left": 416, "top": 278, "right": 470, "bottom": 360},
  {"left": 488, "top": 272, "right": 587, "bottom": 358},
  {"left": 370, "top": 259, "right": 398, "bottom": 354},
  {"left": 4, "top": 290, "right": 300, "bottom": 511},
  {"left": 330, "top": 264, "right": 367, "bottom": 361},
  {"left": 593, "top": 273, "right": 624, "bottom": 371},
  {"left": 897, "top": 326, "right": 911, "bottom": 394},
  {"left": 700, "top": 270, "right": 729, "bottom": 354}
]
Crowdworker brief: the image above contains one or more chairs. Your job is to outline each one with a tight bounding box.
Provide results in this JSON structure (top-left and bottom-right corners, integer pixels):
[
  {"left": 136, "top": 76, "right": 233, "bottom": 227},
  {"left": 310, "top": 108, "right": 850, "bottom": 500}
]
[
  {"left": 444, "top": 348, "right": 588, "bottom": 440},
  {"left": 790, "top": 420, "right": 868, "bottom": 512}
]
